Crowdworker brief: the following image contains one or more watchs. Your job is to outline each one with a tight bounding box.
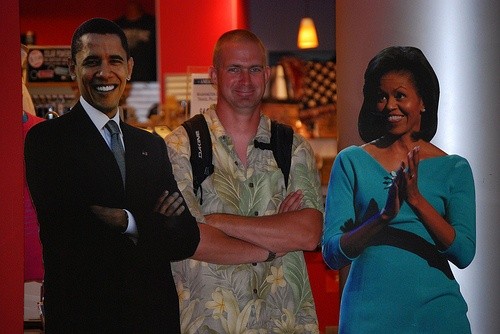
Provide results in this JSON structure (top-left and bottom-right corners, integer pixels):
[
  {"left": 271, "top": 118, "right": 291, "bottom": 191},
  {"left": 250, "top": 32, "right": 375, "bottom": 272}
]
[{"left": 262, "top": 251, "right": 276, "bottom": 263}]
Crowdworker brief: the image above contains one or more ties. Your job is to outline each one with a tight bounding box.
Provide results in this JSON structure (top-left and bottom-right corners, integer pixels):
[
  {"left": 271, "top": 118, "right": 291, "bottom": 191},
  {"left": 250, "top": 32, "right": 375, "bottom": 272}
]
[{"left": 104, "top": 120, "right": 126, "bottom": 192}]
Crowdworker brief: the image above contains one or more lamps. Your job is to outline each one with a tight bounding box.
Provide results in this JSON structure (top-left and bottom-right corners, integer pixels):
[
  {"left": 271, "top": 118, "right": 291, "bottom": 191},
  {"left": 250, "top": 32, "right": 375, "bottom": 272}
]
[{"left": 296, "top": 0, "right": 319, "bottom": 48}]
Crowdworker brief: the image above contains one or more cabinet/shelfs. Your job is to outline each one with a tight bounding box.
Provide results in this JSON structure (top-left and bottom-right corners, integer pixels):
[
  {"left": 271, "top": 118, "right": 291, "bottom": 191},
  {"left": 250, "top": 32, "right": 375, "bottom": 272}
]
[{"left": 21, "top": 42, "right": 81, "bottom": 120}]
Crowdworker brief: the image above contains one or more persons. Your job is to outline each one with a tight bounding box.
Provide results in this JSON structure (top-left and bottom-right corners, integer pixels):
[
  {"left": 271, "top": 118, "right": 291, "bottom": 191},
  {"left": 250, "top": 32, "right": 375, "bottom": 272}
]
[
  {"left": 23, "top": 19, "right": 200, "bottom": 334},
  {"left": 320, "top": 46, "right": 478, "bottom": 334},
  {"left": 165, "top": 28, "right": 319, "bottom": 334}
]
